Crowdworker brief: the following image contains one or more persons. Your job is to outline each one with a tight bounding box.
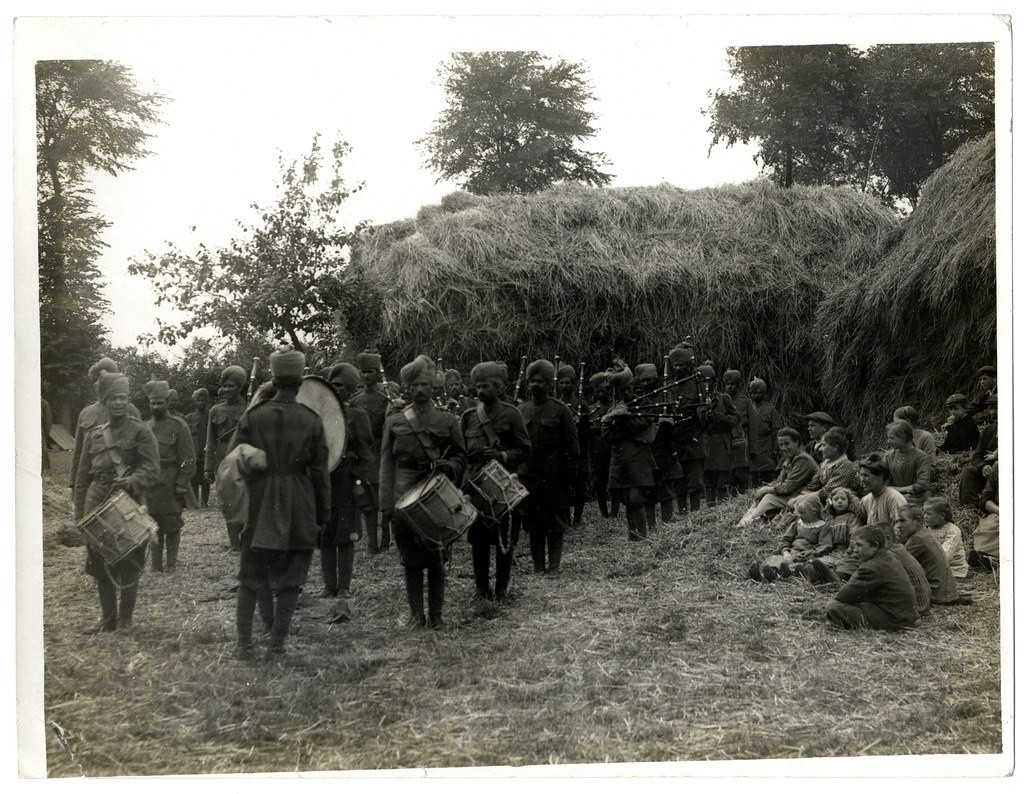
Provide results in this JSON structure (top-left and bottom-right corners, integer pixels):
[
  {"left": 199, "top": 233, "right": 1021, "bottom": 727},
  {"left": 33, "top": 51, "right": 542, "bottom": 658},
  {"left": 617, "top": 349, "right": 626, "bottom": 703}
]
[
  {"left": 731, "top": 365, "right": 999, "bottom": 629},
  {"left": 215, "top": 350, "right": 327, "bottom": 659},
  {"left": 41, "top": 398, "right": 52, "bottom": 469},
  {"left": 73, "top": 373, "right": 161, "bottom": 637},
  {"left": 70, "top": 342, "right": 778, "bottom": 632}
]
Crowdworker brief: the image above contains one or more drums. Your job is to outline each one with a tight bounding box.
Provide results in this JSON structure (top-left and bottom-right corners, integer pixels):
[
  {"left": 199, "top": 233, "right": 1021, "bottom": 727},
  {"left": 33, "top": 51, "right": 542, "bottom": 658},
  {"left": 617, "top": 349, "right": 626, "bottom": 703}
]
[
  {"left": 77, "top": 490, "right": 160, "bottom": 565},
  {"left": 462, "top": 458, "right": 529, "bottom": 528},
  {"left": 249, "top": 375, "right": 347, "bottom": 473},
  {"left": 395, "top": 468, "right": 478, "bottom": 550}
]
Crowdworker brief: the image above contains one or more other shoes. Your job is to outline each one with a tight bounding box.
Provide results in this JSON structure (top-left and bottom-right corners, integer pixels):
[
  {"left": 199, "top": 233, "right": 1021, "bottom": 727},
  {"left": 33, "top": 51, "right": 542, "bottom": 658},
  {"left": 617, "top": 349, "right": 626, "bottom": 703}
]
[
  {"left": 265, "top": 645, "right": 309, "bottom": 662},
  {"left": 968, "top": 549, "right": 992, "bottom": 573},
  {"left": 232, "top": 643, "right": 265, "bottom": 660},
  {"left": 749, "top": 559, "right": 840, "bottom": 584},
  {"left": 81, "top": 616, "right": 137, "bottom": 635},
  {"left": 367, "top": 544, "right": 389, "bottom": 554},
  {"left": 313, "top": 589, "right": 350, "bottom": 599},
  {"left": 404, "top": 614, "right": 447, "bottom": 630}
]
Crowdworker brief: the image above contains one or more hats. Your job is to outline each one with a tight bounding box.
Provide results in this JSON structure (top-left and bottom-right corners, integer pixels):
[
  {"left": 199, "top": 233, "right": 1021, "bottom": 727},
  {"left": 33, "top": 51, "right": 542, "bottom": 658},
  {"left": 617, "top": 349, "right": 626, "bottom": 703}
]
[
  {"left": 750, "top": 376, "right": 766, "bottom": 392},
  {"left": 804, "top": 412, "right": 834, "bottom": 424},
  {"left": 985, "top": 395, "right": 997, "bottom": 404},
  {"left": 590, "top": 358, "right": 657, "bottom": 392},
  {"left": 142, "top": 380, "right": 179, "bottom": 401},
  {"left": 697, "top": 360, "right": 715, "bottom": 378},
  {"left": 471, "top": 361, "right": 509, "bottom": 386},
  {"left": 526, "top": 358, "right": 577, "bottom": 381},
  {"left": 976, "top": 366, "right": 995, "bottom": 377},
  {"left": 946, "top": 394, "right": 969, "bottom": 407},
  {"left": 216, "top": 365, "right": 247, "bottom": 397},
  {"left": 192, "top": 388, "right": 208, "bottom": 401},
  {"left": 88, "top": 357, "right": 130, "bottom": 402},
  {"left": 270, "top": 345, "right": 305, "bottom": 377},
  {"left": 433, "top": 368, "right": 462, "bottom": 387},
  {"left": 668, "top": 341, "right": 695, "bottom": 363},
  {"left": 400, "top": 355, "right": 436, "bottom": 386},
  {"left": 357, "top": 348, "right": 381, "bottom": 369},
  {"left": 723, "top": 368, "right": 740, "bottom": 384},
  {"left": 319, "top": 364, "right": 360, "bottom": 386},
  {"left": 387, "top": 381, "right": 400, "bottom": 394}
]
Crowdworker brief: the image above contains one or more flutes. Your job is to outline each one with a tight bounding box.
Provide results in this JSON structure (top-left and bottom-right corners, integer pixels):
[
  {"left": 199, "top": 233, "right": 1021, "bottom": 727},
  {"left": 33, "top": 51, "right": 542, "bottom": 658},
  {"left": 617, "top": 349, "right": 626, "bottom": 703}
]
[{"left": 433, "top": 354, "right": 718, "bottom": 424}]
